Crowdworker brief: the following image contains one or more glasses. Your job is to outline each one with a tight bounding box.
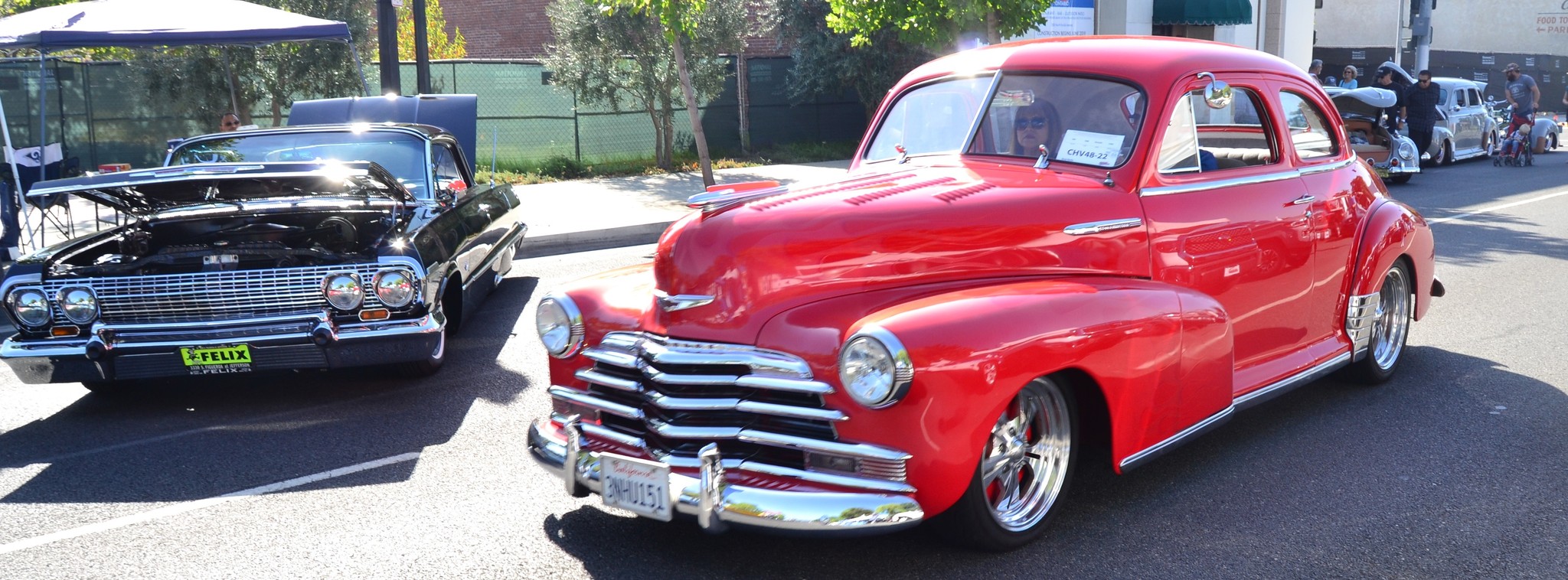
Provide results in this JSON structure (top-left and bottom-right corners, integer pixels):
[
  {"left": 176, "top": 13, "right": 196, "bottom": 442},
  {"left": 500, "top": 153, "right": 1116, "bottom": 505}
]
[
  {"left": 221, "top": 121, "right": 240, "bottom": 126},
  {"left": 1014, "top": 117, "right": 1048, "bottom": 131},
  {"left": 1377, "top": 76, "right": 1384, "bottom": 78},
  {"left": 1418, "top": 77, "right": 1430, "bottom": 82},
  {"left": 1129, "top": 115, "right": 1142, "bottom": 124},
  {"left": 1345, "top": 71, "right": 1351, "bottom": 74}
]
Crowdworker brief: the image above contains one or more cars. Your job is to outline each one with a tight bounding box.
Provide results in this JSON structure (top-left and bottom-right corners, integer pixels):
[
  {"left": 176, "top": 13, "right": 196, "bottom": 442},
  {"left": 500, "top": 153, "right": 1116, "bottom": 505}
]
[
  {"left": 1323, "top": 86, "right": 1421, "bottom": 183},
  {"left": 1378, "top": 61, "right": 1501, "bottom": 168},
  {"left": 0, "top": 94, "right": 528, "bottom": 400},
  {"left": 526, "top": 35, "right": 1447, "bottom": 554}
]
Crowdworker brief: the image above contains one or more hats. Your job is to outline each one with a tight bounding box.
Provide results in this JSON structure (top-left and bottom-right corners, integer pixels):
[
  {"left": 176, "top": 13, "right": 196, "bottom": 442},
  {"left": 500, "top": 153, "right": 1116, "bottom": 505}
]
[
  {"left": 1325, "top": 76, "right": 1337, "bottom": 86},
  {"left": 1375, "top": 66, "right": 1390, "bottom": 77},
  {"left": 1501, "top": 63, "right": 1520, "bottom": 73}
]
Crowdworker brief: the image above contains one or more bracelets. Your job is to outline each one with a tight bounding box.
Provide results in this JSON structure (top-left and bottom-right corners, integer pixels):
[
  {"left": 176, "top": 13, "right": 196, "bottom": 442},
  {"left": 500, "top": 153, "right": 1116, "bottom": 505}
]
[{"left": 1399, "top": 119, "right": 1406, "bottom": 123}]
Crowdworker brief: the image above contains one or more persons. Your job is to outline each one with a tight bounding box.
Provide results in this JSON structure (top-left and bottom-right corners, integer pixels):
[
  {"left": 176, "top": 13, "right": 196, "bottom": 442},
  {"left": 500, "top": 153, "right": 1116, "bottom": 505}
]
[
  {"left": 1323, "top": 76, "right": 1337, "bottom": 87},
  {"left": 1562, "top": 85, "right": 1568, "bottom": 126},
  {"left": 1505, "top": 63, "right": 1540, "bottom": 161},
  {"left": 1007, "top": 98, "right": 1063, "bottom": 159},
  {"left": 219, "top": 112, "right": 241, "bottom": 132},
  {"left": 1407, "top": 70, "right": 1440, "bottom": 174},
  {"left": 1308, "top": 59, "right": 1323, "bottom": 86},
  {"left": 1370, "top": 66, "right": 1407, "bottom": 135},
  {"left": 1338, "top": 65, "right": 1358, "bottom": 90},
  {"left": 1499, "top": 124, "right": 1531, "bottom": 158},
  {"left": 1133, "top": 94, "right": 1220, "bottom": 176}
]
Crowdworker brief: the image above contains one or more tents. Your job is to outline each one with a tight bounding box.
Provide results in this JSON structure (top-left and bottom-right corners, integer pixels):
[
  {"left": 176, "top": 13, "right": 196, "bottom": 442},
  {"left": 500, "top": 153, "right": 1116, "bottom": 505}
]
[{"left": 0, "top": 0, "right": 371, "bottom": 251}]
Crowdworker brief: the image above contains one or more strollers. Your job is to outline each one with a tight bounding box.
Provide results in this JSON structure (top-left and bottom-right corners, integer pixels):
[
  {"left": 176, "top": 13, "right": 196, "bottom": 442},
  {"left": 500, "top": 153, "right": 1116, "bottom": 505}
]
[{"left": 1493, "top": 107, "right": 1538, "bottom": 168}]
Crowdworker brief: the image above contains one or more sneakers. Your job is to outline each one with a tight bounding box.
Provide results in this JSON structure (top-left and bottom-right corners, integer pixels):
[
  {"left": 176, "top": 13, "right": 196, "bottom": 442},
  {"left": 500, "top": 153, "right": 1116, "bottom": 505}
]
[
  {"left": 1509, "top": 152, "right": 1515, "bottom": 158},
  {"left": 1499, "top": 152, "right": 1505, "bottom": 157}
]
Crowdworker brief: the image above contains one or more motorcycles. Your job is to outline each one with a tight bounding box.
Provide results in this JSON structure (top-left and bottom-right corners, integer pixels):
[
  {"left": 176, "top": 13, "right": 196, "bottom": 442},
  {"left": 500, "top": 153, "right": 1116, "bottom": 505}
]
[{"left": 1484, "top": 95, "right": 1563, "bottom": 156}]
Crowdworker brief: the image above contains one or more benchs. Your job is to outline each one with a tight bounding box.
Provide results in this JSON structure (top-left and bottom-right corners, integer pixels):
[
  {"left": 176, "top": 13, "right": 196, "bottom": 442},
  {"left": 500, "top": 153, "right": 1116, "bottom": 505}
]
[{"left": 1198, "top": 144, "right": 1327, "bottom": 166}]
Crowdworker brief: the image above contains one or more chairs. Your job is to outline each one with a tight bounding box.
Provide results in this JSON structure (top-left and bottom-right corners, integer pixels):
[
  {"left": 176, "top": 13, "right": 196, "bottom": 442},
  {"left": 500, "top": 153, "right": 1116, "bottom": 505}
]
[
  {"left": 2, "top": 141, "right": 76, "bottom": 257},
  {"left": 1216, "top": 157, "right": 1247, "bottom": 170}
]
